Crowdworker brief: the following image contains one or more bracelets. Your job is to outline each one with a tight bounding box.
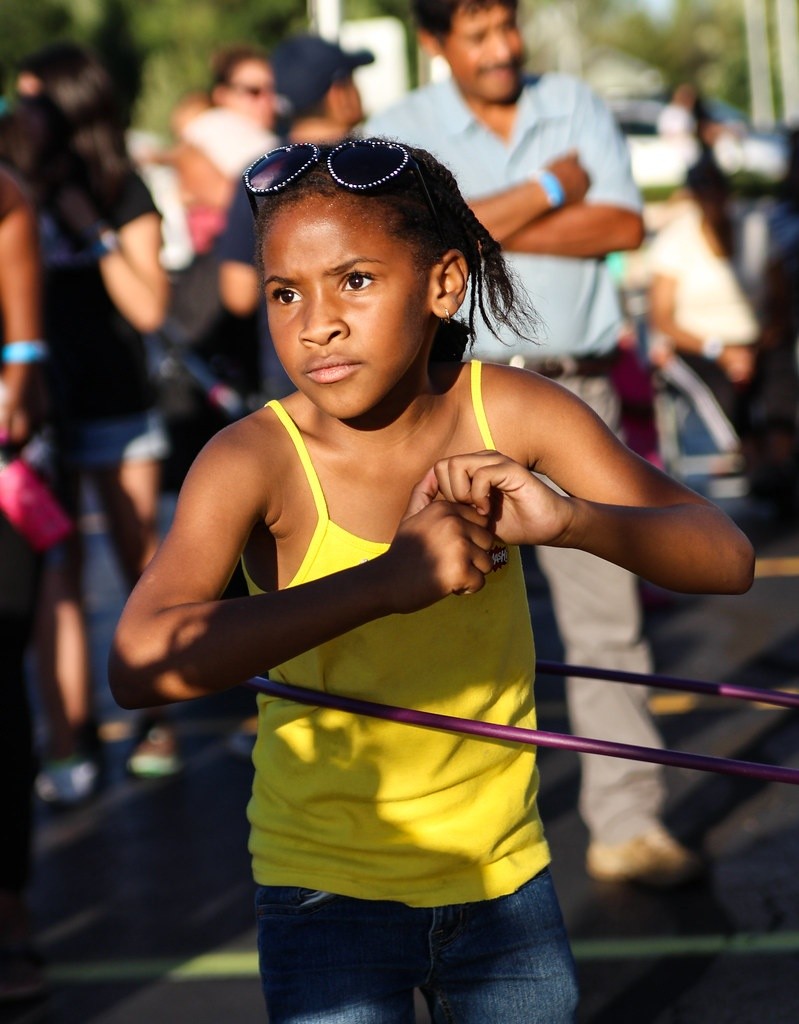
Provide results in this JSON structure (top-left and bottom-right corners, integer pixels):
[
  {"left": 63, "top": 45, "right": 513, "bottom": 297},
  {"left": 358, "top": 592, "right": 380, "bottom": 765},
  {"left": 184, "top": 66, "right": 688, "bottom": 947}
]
[
  {"left": 533, "top": 170, "right": 565, "bottom": 209},
  {"left": 2, "top": 340, "right": 48, "bottom": 364}
]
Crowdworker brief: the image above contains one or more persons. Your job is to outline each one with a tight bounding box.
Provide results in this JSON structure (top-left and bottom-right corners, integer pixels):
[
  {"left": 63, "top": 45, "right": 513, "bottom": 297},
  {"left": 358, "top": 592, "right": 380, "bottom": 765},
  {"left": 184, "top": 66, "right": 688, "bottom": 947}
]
[{"left": 0, "top": 0, "right": 799, "bottom": 1024}]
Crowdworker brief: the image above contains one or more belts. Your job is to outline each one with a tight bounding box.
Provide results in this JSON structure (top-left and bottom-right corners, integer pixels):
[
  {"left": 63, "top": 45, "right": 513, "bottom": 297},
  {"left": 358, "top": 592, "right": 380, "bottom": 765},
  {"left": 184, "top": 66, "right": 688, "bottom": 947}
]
[{"left": 497, "top": 360, "right": 620, "bottom": 379}]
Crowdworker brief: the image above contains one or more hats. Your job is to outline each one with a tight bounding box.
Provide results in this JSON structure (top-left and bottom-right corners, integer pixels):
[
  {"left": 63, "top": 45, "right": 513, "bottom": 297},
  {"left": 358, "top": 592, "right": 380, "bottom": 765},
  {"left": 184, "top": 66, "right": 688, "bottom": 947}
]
[{"left": 272, "top": 39, "right": 375, "bottom": 114}]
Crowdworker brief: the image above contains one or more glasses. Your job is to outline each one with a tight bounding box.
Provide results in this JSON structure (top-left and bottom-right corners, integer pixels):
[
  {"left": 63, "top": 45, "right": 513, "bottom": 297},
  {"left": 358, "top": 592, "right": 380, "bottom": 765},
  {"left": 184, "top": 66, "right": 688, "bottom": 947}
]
[{"left": 241, "top": 140, "right": 439, "bottom": 221}]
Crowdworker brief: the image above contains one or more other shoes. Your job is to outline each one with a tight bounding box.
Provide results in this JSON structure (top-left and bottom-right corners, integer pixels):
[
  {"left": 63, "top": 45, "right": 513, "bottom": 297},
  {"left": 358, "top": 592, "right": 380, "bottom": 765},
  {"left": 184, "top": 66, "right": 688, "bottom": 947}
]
[
  {"left": 125, "top": 732, "right": 187, "bottom": 779},
  {"left": 35, "top": 753, "right": 97, "bottom": 802},
  {"left": 586, "top": 826, "right": 692, "bottom": 885}
]
